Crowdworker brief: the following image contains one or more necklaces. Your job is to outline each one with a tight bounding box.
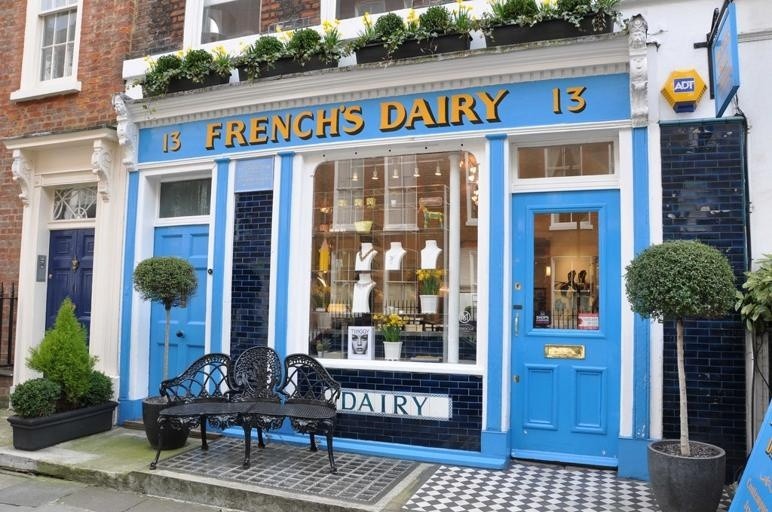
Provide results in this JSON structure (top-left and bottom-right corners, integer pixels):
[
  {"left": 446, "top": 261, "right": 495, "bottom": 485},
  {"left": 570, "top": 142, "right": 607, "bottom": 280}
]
[
  {"left": 359, "top": 248, "right": 375, "bottom": 261},
  {"left": 357, "top": 279, "right": 375, "bottom": 289}
]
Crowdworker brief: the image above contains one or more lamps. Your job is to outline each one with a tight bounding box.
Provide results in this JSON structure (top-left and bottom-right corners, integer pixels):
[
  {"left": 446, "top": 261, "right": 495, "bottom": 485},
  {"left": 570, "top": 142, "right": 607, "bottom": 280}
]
[{"left": 348, "top": 154, "right": 444, "bottom": 183}]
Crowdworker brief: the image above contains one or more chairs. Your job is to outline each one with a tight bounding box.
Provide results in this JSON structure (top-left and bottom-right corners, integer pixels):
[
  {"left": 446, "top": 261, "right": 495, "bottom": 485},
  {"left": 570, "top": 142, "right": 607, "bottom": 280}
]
[{"left": 148, "top": 344, "right": 344, "bottom": 473}]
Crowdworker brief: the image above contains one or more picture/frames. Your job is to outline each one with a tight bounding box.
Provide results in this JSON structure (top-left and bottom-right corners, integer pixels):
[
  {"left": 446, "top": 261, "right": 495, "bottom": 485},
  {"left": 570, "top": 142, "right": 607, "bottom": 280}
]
[{"left": 346, "top": 325, "right": 375, "bottom": 360}]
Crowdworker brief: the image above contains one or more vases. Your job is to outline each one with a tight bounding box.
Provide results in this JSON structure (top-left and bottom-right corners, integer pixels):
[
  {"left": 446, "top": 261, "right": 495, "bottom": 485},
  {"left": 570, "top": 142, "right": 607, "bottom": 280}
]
[
  {"left": 419, "top": 295, "right": 440, "bottom": 314},
  {"left": 382, "top": 341, "right": 404, "bottom": 361}
]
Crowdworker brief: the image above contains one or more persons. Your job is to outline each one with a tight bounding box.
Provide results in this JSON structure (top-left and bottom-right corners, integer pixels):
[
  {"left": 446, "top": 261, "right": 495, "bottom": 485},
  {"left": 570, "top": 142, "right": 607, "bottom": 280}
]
[{"left": 352, "top": 331, "right": 369, "bottom": 355}]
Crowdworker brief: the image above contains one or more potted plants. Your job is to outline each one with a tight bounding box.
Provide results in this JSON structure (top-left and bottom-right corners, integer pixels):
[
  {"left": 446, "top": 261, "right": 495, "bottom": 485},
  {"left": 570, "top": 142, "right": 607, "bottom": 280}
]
[
  {"left": 621, "top": 235, "right": 738, "bottom": 511},
  {"left": 4, "top": 295, "right": 121, "bottom": 454},
  {"left": 131, "top": 256, "right": 197, "bottom": 452},
  {"left": 316, "top": 338, "right": 332, "bottom": 358}
]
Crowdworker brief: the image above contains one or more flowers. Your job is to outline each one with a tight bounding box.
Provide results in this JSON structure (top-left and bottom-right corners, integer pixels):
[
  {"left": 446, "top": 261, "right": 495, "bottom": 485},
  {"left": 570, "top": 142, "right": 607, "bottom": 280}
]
[
  {"left": 414, "top": 267, "right": 445, "bottom": 295},
  {"left": 373, "top": 313, "right": 405, "bottom": 341}
]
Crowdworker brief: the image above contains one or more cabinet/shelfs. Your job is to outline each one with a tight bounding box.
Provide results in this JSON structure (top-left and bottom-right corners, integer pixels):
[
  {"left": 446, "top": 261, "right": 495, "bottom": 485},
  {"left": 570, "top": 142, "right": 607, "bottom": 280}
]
[{"left": 314, "top": 182, "right": 450, "bottom": 364}]
